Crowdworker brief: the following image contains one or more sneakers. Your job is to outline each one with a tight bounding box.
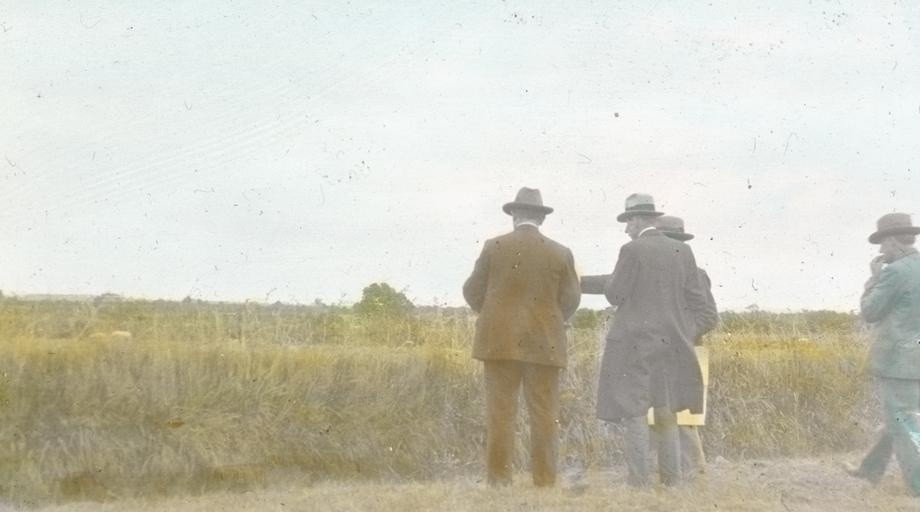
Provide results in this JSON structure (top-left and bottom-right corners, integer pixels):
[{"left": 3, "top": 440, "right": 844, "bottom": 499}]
[{"left": 843, "top": 461, "right": 881, "bottom": 488}]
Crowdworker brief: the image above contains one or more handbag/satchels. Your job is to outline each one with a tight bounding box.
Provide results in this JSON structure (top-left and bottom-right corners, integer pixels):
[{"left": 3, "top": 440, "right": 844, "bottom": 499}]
[{"left": 647, "top": 346, "right": 708, "bottom": 425}]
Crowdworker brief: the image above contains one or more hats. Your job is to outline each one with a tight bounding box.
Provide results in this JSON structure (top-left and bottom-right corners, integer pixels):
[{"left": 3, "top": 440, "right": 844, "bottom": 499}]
[
  {"left": 503, "top": 186, "right": 553, "bottom": 216},
  {"left": 868, "top": 213, "right": 920, "bottom": 242},
  {"left": 654, "top": 215, "right": 694, "bottom": 241},
  {"left": 617, "top": 193, "right": 665, "bottom": 224}
]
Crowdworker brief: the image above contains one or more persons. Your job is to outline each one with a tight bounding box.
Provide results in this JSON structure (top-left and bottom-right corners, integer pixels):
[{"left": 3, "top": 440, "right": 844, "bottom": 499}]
[
  {"left": 463, "top": 188, "right": 581, "bottom": 487},
  {"left": 838, "top": 210, "right": 920, "bottom": 498},
  {"left": 577, "top": 215, "right": 721, "bottom": 478},
  {"left": 594, "top": 191, "right": 707, "bottom": 489}
]
